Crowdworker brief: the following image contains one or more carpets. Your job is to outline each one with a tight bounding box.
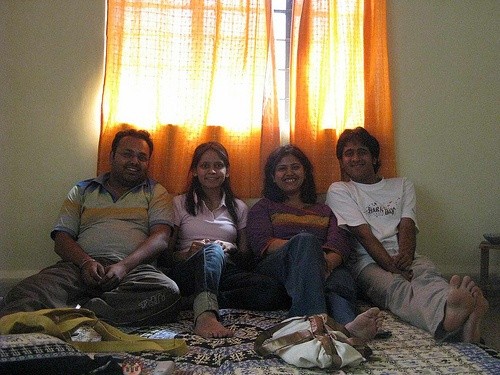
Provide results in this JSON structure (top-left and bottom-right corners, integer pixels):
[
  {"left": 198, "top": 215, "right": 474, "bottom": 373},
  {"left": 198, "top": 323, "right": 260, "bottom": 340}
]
[{"left": 71, "top": 303, "right": 500, "bottom": 375}]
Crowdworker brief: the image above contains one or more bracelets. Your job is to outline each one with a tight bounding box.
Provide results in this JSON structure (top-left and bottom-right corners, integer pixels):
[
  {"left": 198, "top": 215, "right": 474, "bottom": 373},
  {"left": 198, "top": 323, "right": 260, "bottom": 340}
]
[{"left": 79, "top": 257, "right": 96, "bottom": 268}]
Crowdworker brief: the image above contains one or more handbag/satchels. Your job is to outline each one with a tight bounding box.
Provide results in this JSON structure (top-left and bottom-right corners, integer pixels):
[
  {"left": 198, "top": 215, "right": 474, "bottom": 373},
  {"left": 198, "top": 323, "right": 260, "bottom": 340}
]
[{"left": 253, "top": 312, "right": 373, "bottom": 369}]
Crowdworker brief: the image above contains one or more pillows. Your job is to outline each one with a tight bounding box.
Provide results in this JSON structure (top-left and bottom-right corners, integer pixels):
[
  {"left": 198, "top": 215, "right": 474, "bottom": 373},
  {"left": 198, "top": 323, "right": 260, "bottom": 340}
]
[{"left": 0, "top": 333, "right": 98, "bottom": 375}]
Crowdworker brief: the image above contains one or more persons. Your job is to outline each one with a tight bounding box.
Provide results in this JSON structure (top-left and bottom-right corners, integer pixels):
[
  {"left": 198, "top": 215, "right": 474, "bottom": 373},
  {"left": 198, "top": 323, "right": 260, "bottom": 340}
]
[
  {"left": 157, "top": 140, "right": 249, "bottom": 340},
  {"left": 238, "top": 144, "right": 385, "bottom": 342},
  {"left": 325, "top": 126, "right": 489, "bottom": 345},
  {"left": 0, "top": 127, "right": 181, "bottom": 333}
]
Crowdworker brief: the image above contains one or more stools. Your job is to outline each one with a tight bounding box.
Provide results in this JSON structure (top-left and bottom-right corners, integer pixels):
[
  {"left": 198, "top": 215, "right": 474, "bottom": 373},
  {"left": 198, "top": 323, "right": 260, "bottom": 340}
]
[{"left": 478, "top": 241, "right": 500, "bottom": 297}]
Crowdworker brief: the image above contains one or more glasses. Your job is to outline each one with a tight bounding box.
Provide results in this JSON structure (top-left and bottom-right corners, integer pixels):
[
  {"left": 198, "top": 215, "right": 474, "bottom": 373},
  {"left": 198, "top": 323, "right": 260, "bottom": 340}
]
[{"left": 116, "top": 151, "right": 148, "bottom": 162}]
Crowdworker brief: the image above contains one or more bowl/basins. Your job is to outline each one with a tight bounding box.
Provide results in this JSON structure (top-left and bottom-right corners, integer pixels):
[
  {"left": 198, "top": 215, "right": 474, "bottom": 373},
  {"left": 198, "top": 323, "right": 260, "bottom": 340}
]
[{"left": 483, "top": 233, "right": 500, "bottom": 244}]
